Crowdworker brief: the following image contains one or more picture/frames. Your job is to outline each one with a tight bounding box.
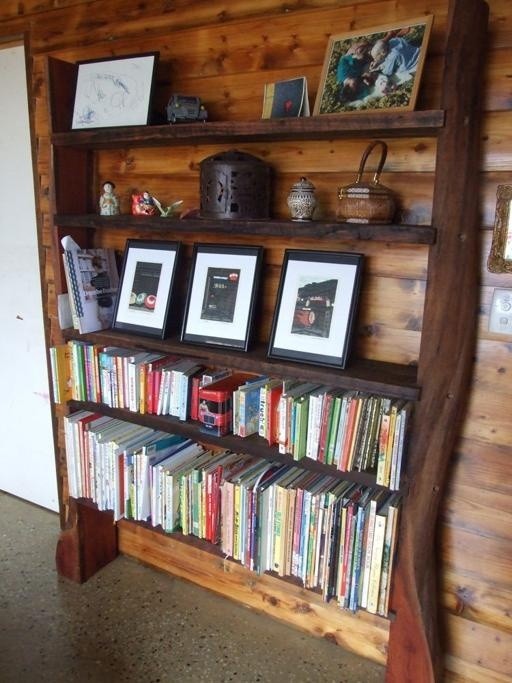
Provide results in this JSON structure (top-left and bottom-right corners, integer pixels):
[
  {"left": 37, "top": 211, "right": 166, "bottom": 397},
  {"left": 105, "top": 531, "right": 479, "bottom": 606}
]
[
  {"left": 112, "top": 238, "right": 181, "bottom": 341},
  {"left": 70, "top": 50, "right": 160, "bottom": 132},
  {"left": 181, "top": 242, "right": 259, "bottom": 353},
  {"left": 266, "top": 248, "right": 365, "bottom": 371},
  {"left": 310, "top": 12, "right": 434, "bottom": 118}
]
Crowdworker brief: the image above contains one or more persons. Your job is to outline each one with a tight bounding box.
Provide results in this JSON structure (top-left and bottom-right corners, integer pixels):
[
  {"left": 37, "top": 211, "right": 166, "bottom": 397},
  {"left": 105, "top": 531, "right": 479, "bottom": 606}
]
[
  {"left": 371, "top": 34, "right": 421, "bottom": 91},
  {"left": 99, "top": 180, "right": 121, "bottom": 218},
  {"left": 336, "top": 39, "right": 366, "bottom": 101},
  {"left": 142, "top": 189, "right": 154, "bottom": 207},
  {"left": 83, "top": 256, "right": 114, "bottom": 329}
]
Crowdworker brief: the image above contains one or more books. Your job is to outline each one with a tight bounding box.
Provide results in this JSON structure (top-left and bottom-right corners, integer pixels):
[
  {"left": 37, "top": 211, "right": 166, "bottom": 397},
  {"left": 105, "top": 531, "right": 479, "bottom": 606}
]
[
  {"left": 47, "top": 339, "right": 229, "bottom": 422},
  {"left": 229, "top": 374, "right": 415, "bottom": 491},
  {"left": 61, "top": 246, "right": 120, "bottom": 336},
  {"left": 62, "top": 411, "right": 403, "bottom": 615}
]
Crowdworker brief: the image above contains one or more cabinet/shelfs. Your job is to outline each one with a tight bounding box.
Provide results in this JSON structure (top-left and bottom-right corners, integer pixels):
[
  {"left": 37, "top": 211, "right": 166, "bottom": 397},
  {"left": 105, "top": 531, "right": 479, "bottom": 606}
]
[{"left": 18, "top": 2, "right": 491, "bottom": 682}]
